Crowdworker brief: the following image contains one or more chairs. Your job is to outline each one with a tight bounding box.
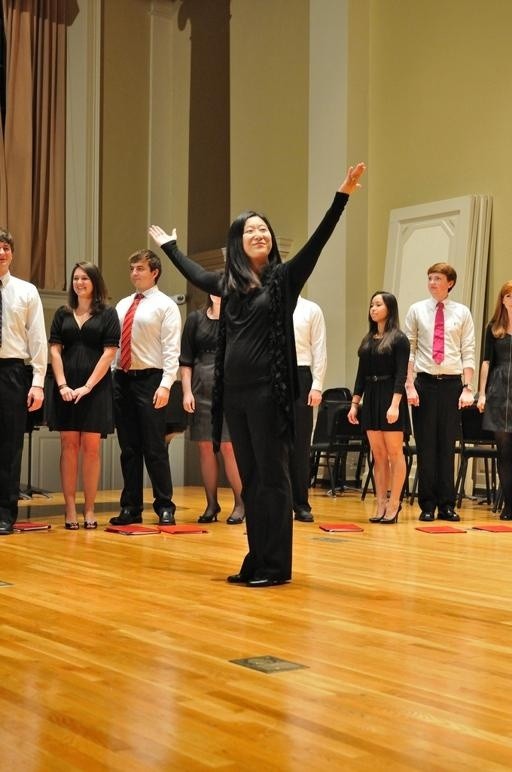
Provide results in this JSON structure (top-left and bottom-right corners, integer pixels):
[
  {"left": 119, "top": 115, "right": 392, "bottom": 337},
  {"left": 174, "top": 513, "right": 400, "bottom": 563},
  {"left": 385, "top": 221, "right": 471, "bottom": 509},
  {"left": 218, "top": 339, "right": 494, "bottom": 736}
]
[{"left": 309, "top": 389, "right": 500, "bottom": 512}]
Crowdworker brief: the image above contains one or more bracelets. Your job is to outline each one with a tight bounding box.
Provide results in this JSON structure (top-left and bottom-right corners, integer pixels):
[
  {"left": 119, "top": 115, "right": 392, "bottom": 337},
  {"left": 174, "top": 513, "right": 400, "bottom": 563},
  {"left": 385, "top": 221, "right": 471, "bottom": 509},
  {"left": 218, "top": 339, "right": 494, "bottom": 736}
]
[
  {"left": 58, "top": 384, "right": 68, "bottom": 391},
  {"left": 351, "top": 402, "right": 359, "bottom": 405},
  {"left": 83, "top": 384, "right": 91, "bottom": 391}
]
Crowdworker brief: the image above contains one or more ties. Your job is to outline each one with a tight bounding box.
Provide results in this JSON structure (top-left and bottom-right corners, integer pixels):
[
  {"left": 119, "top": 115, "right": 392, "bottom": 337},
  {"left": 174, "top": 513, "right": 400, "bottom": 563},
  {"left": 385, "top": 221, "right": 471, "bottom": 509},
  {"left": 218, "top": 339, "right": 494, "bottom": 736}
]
[
  {"left": 120, "top": 294, "right": 145, "bottom": 372},
  {"left": 432, "top": 302, "right": 444, "bottom": 366}
]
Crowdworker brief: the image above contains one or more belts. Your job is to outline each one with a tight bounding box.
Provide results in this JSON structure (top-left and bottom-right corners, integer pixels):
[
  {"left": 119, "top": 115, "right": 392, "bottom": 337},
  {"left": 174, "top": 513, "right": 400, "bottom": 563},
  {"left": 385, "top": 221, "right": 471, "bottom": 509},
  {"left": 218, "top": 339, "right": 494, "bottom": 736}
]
[
  {"left": 366, "top": 375, "right": 391, "bottom": 382},
  {"left": 417, "top": 372, "right": 462, "bottom": 380}
]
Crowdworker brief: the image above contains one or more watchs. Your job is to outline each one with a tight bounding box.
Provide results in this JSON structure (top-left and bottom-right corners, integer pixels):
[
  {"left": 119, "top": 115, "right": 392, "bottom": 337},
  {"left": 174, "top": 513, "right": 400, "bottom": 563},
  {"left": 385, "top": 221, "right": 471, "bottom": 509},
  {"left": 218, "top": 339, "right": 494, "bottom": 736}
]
[{"left": 462, "top": 383, "right": 473, "bottom": 391}]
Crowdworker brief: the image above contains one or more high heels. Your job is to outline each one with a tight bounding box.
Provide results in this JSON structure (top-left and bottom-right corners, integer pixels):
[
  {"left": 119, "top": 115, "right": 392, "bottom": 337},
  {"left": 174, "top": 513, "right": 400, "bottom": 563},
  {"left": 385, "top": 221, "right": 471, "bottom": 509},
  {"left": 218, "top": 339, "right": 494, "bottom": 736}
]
[
  {"left": 380, "top": 503, "right": 402, "bottom": 523},
  {"left": 82, "top": 510, "right": 97, "bottom": 529},
  {"left": 64, "top": 513, "right": 80, "bottom": 529},
  {"left": 226, "top": 509, "right": 247, "bottom": 523},
  {"left": 198, "top": 505, "right": 221, "bottom": 523},
  {"left": 369, "top": 507, "right": 385, "bottom": 522}
]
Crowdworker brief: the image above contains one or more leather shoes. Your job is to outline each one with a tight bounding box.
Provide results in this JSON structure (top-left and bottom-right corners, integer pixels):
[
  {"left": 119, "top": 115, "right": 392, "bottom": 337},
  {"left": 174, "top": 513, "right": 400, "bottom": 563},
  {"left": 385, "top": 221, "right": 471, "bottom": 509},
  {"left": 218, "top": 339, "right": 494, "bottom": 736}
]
[
  {"left": 228, "top": 570, "right": 255, "bottom": 582},
  {"left": 499, "top": 506, "right": 511, "bottom": 520},
  {"left": 295, "top": 507, "right": 314, "bottom": 522},
  {"left": 247, "top": 569, "right": 290, "bottom": 584},
  {"left": 1, "top": 518, "right": 15, "bottom": 535},
  {"left": 159, "top": 509, "right": 176, "bottom": 525},
  {"left": 109, "top": 507, "right": 143, "bottom": 525},
  {"left": 437, "top": 506, "right": 460, "bottom": 521},
  {"left": 418, "top": 510, "right": 434, "bottom": 521}
]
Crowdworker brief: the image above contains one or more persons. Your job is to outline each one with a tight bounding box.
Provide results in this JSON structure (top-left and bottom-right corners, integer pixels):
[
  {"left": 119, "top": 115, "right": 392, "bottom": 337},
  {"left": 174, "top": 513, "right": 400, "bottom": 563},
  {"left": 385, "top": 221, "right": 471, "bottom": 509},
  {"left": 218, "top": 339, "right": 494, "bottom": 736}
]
[
  {"left": 347, "top": 291, "right": 411, "bottom": 524},
  {"left": 405, "top": 263, "right": 476, "bottom": 521},
  {"left": 292, "top": 295, "right": 329, "bottom": 522},
  {"left": 49, "top": 261, "right": 122, "bottom": 530},
  {"left": 148, "top": 161, "right": 367, "bottom": 586},
  {"left": 178, "top": 268, "right": 247, "bottom": 525},
  {"left": 108, "top": 249, "right": 182, "bottom": 525},
  {"left": 477, "top": 280, "right": 511, "bottom": 520},
  {"left": 0, "top": 227, "right": 50, "bottom": 535}
]
